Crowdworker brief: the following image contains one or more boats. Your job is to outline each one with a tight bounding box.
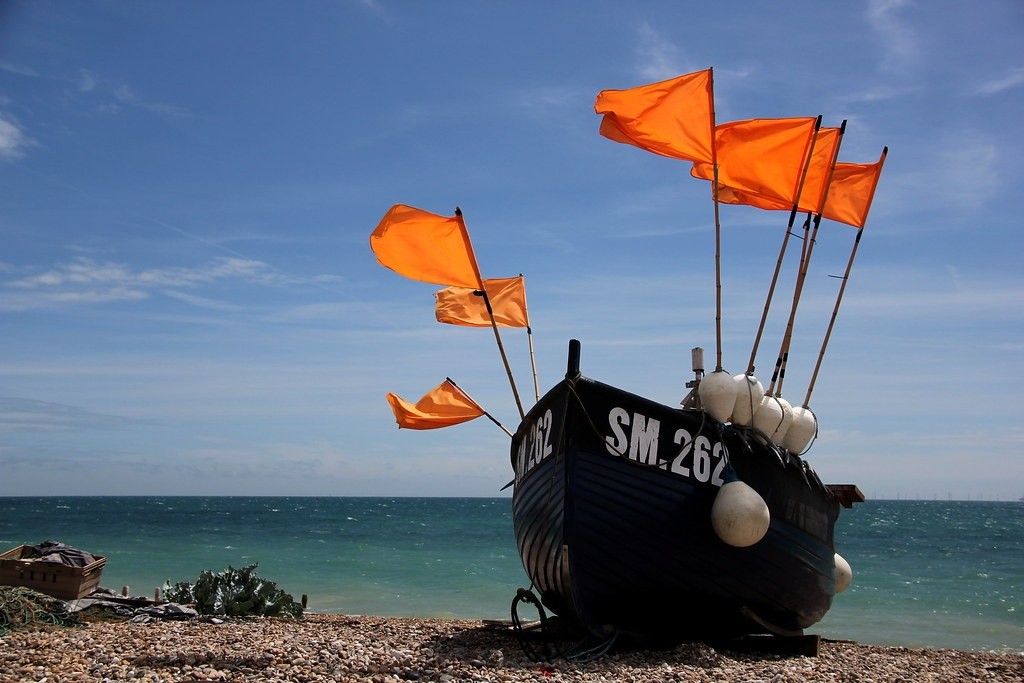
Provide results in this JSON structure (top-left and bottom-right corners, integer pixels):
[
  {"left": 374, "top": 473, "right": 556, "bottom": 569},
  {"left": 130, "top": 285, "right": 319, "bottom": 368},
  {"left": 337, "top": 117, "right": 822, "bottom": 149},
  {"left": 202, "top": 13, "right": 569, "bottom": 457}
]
[{"left": 509, "top": 340, "right": 866, "bottom": 633}]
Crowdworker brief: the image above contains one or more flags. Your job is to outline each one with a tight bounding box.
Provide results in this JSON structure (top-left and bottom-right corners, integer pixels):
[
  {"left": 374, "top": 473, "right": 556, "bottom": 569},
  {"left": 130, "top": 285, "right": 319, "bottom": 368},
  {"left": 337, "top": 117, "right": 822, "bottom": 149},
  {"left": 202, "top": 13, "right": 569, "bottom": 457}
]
[
  {"left": 821, "top": 154, "right": 884, "bottom": 229},
  {"left": 594, "top": 68, "right": 714, "bottom": 165},
  {"left": 370, "top": 203, "right": 485, "bottom": 289},
  {"left": 386, "top": 380, "right": 485, "bottom": 430},
  {"left": 435, "top": 276, "right": 529, "bottom": 326},
  {"left": 692, "top": 116, "right": 818, "bottom": 204},
  {"left": 711, "top": 127, "right": 842, "bottom": 218}
]
